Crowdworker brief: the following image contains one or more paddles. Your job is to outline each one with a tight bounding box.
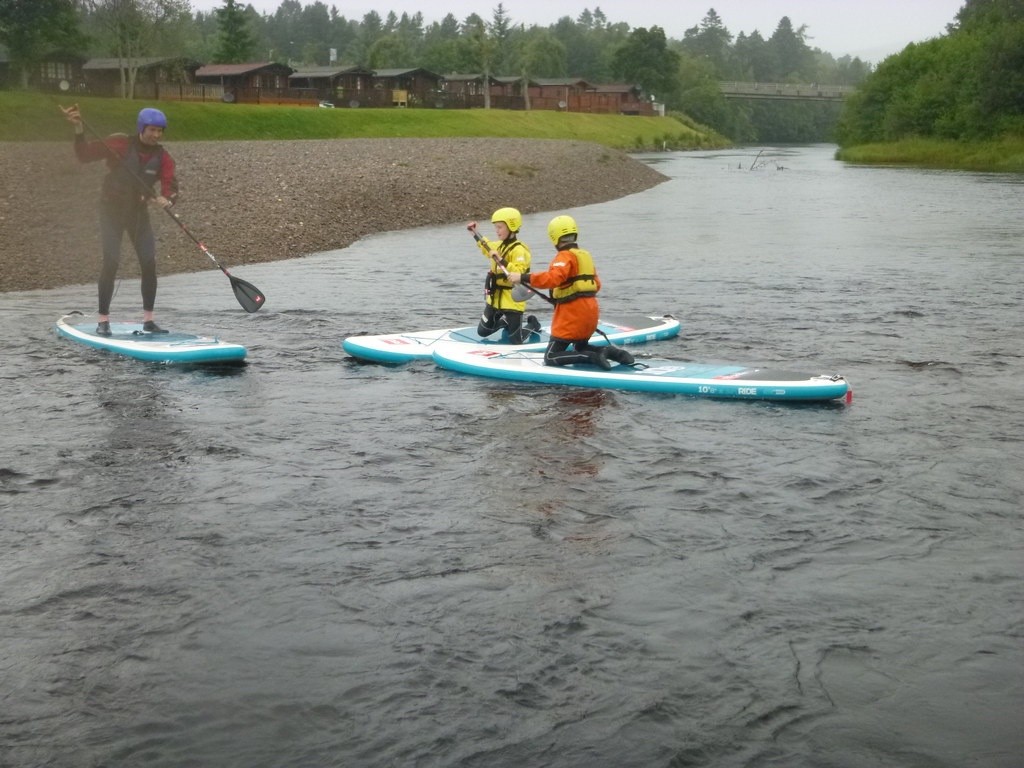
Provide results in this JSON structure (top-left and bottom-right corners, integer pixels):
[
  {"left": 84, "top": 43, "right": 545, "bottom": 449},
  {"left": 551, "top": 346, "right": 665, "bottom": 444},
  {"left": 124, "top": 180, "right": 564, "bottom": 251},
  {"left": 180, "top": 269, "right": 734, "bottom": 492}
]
[
  {"left": 468, "top": 223, "right": 539, "bottom": 303},
  {"left": 75, "top": 111, "right": 268, "bottom": 318},
  {"left": 515, "top": 278, "right": 621, "bottom": 351}
]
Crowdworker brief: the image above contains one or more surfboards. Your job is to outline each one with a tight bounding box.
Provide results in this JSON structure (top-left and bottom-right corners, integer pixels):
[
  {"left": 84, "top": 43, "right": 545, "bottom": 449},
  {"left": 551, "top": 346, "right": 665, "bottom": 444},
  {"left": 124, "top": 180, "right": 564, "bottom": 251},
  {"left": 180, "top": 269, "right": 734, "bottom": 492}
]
[
  {"left": 431, "top": 341, "right": 851, "bottom": 405},
  {"left": 341, "top": 312, "right": 683, "bottom": 366},
  {"left": 56, "top": 308, "right": 248, "bottom": 366}
]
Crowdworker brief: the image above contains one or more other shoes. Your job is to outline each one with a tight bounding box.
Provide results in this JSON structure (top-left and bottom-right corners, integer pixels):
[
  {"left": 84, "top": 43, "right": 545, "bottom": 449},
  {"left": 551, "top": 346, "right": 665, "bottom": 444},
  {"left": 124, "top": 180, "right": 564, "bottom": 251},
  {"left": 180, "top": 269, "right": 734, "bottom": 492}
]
[
  {"left": 528, "top": 315, "right": 541, "bottom": 330},
  {"left": 605, "top": 346, "right": 634, "bottom": 364},
  {"left": 143, "top": 321, "right": 169, "bottom": 333},
  {"left": 593, "top": 352, "right": 612, "bottom": 371},
  {"left": 96, "top": 321, "right": 112, "bottom": 335}
]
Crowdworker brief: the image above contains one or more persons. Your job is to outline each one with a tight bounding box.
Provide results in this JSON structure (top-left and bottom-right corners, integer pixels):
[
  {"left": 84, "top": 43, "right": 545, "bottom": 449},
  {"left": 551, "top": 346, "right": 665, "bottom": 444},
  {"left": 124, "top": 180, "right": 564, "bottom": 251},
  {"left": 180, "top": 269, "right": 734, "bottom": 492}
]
[
  {"left": 466, "top": 207, "right": 541, "bottom": 344},
  {"left": 58, "top": 103, "right": 179, "bottom": 336},
  {"left": 508, "top": 215, "right": 635, "bottom": 370}
]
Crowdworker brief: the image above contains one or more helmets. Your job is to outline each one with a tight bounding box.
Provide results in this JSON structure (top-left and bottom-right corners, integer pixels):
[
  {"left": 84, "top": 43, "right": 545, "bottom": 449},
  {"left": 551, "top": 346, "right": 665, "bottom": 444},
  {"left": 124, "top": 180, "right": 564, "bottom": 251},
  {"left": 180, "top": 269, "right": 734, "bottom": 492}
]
[
  {"left": 491, "top": 208, "right": 522, "bottom": 232},
  {"left": 136, "top": 108, "right": 166, "bottom": 133},
  {"left": 547, "top": 215, "right": 578, "bottom": 246}
]
